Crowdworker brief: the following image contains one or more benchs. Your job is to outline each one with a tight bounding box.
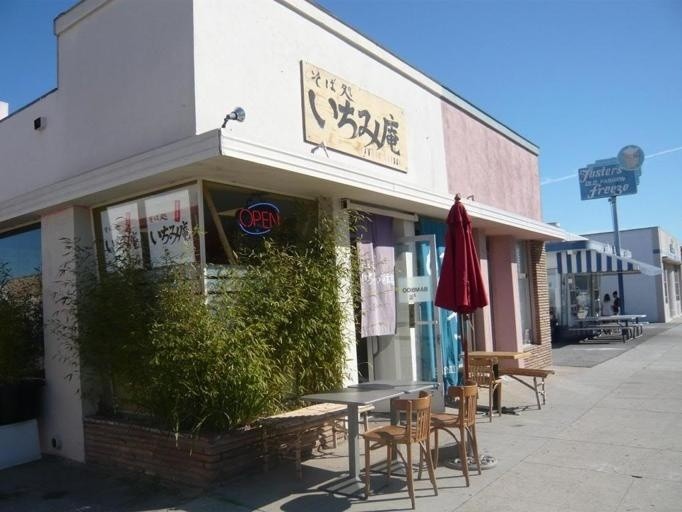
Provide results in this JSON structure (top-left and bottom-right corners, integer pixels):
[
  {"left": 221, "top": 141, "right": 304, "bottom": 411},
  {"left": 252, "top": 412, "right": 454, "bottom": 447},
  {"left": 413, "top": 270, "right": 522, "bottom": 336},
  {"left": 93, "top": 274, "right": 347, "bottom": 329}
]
[
  {"left": 459, "top": 365, "right": 555, "bottom": 409},
  {"left": 567, "top": 321, "right": 649, "bottom": 343},
  {"left": 257, "top": 402, "right": 375, "bottom": 483}
]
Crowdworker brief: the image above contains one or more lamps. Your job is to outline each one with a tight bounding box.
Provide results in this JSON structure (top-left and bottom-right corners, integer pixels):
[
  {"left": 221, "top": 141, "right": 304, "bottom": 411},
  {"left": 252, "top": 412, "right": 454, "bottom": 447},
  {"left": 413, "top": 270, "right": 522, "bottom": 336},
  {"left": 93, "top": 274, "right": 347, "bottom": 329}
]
[{"left": 220, "top": 108, "right": 246, "bottom": 129}]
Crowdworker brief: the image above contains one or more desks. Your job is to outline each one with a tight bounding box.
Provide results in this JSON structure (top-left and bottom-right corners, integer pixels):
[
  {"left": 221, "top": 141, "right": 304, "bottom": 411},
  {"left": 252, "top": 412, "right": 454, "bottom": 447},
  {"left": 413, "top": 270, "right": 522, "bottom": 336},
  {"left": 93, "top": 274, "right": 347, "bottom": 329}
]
[
  {"left": 298, "top": 379, "right": 439, "bottom": 499},
  {"left": 574, "top": 315, "right": 646, "bottom": 340},
  {"left": 459, "top": 350, "right": 531, "bottom": 414}
]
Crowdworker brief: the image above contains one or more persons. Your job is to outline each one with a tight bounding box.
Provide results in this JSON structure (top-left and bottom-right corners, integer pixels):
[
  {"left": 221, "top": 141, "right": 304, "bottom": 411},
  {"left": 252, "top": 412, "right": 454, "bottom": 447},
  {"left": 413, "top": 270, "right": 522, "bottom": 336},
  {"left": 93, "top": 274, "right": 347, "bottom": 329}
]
[{"left": 602, "top": 291, "right": 621, "bottom": 335}]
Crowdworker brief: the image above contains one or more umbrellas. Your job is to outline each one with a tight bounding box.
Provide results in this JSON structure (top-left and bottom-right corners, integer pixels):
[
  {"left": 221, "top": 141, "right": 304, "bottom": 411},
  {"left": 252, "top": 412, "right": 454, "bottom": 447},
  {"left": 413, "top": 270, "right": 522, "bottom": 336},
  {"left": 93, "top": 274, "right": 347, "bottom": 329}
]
[{"left": 435, "top": 193, "right": 497, "bottom": 471}]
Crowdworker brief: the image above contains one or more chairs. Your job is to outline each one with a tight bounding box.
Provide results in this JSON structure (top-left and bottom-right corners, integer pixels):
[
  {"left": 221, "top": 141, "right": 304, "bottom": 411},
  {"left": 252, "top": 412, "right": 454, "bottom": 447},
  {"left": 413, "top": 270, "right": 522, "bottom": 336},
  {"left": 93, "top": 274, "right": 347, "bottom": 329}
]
[
  {"left": 462, "top": 355, "right": 503, "bottom": 422},
  {"left": 358, "top": 379, "right": 481, "bottom": 508}
]
